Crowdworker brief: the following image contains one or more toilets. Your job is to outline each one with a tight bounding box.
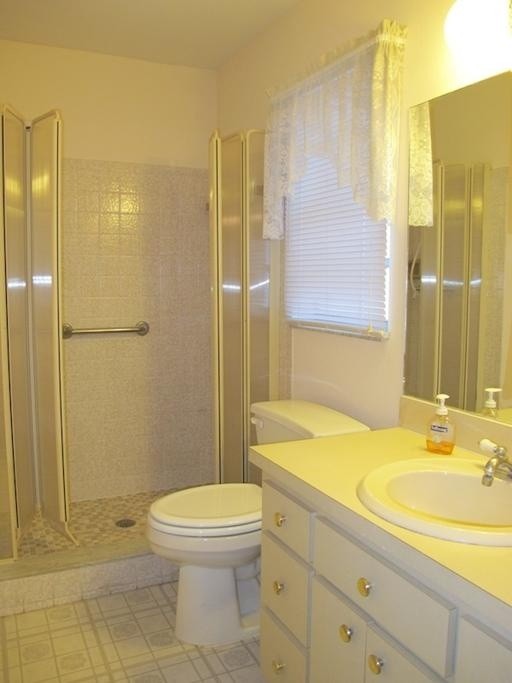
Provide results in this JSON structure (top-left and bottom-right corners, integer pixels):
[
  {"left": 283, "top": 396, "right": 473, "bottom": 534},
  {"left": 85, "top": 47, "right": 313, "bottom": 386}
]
[{"left": 148, "top": 399, "right": 371, "bottom": 645}]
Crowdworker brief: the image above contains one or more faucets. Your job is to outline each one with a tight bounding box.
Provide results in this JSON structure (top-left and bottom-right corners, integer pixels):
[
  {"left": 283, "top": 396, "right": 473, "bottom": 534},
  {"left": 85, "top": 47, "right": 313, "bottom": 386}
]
[{"left": 475, "top": 439, "right": 511, "bottom": 487}]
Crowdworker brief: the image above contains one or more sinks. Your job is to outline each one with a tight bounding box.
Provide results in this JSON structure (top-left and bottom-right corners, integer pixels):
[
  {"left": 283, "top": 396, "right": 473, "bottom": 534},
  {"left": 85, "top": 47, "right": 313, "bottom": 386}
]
[{"left": 357, "top": 457, "right": 511, "bottom": 547}]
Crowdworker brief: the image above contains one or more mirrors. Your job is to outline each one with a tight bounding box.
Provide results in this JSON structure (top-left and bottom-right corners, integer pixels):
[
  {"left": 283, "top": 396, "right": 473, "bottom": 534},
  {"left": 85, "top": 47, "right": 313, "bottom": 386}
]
[{"left": 402, "top": 68, "right": 510, "bottom": 426}]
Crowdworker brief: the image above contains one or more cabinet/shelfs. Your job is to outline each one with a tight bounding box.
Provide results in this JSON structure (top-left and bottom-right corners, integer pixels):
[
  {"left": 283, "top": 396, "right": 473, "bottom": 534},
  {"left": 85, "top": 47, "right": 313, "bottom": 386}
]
[{"left": 258, "top": 466, "right": 512, "bottom": 682}]
